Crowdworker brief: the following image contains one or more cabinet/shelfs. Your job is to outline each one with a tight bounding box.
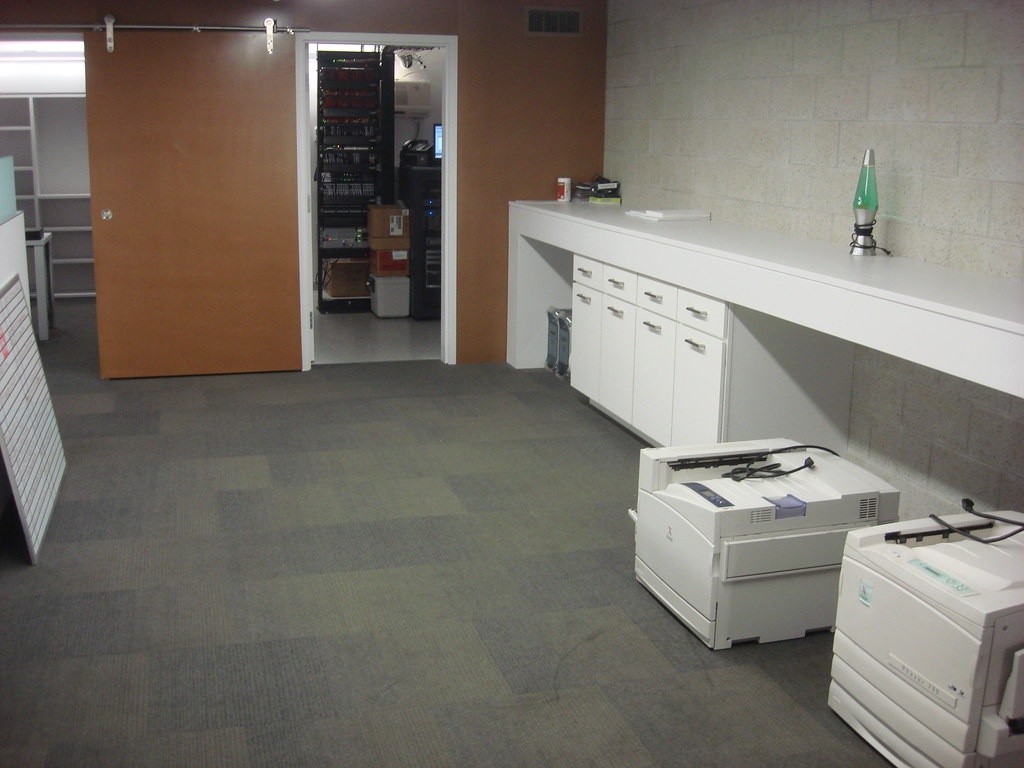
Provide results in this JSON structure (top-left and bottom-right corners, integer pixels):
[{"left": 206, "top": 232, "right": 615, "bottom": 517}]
[
  {"left": 599, "top": 263, "right": 637, "bottom": 425},
  {"left": 671, "top": 288, "right": 854, "bottom": 461},
  {"left": 0, "top": 91, "right": 95, "bottom": 302},
  {"left": 630, "top": 275, "right": 677, "bottom": 449},
  {"left": 567, "top": 253, "right": 601, "bottom": 405},
  {"left": 315, "top": 44, "right": 396, "bottom": 313}
]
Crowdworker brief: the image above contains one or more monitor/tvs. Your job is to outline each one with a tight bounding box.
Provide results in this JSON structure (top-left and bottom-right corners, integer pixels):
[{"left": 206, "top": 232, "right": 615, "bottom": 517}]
[{"left": 433, "top": 124, "right": 443, "bottom": 160}]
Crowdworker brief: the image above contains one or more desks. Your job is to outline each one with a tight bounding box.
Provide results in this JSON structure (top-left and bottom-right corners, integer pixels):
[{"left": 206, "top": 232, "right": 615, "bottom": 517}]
[
  {"left": 506, "top": 199, "right": 1023, "bottom": 400},
  {"left": 25, "top": 231, "right": 54, "bottom": 340}
]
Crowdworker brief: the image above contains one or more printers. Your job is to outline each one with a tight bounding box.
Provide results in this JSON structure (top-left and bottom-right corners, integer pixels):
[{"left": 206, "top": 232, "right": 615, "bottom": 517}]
[
  {"left": 828, "top": 510, "right": 1024, "bottom": 768},
  {"left": 633, "top": 438, "right": 901, "bottom": 651}
]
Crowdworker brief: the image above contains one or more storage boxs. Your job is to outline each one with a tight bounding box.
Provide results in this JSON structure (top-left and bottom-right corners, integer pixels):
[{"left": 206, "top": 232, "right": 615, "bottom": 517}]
[
  {"left": 369, "top": 248, "right": 409, "bottom": 277},
  {"left": 366, "top": 273, "right": 410, "bottom": 319},
  {"left": 325, "top": 260, "right": 370, "bottom": 297},
  {"left": 368, "top": 204, "right": 410, "bottom": 238},
  {"left": 367, "top": 235, "right": 409, "bottom": 251}
]
